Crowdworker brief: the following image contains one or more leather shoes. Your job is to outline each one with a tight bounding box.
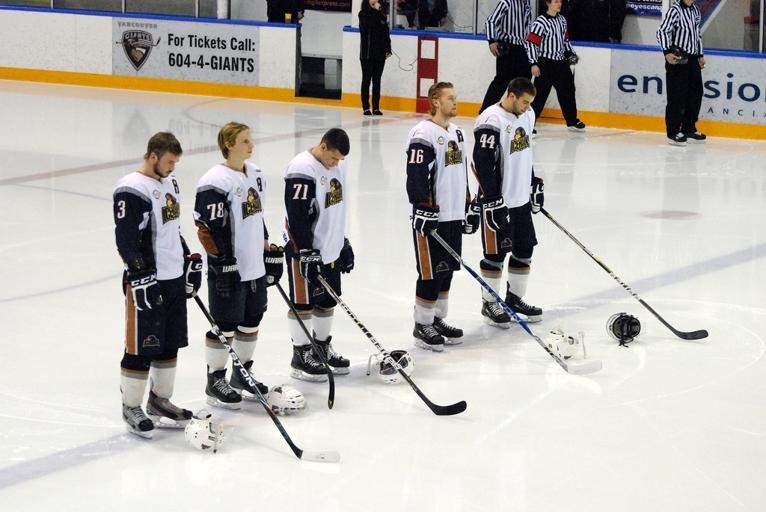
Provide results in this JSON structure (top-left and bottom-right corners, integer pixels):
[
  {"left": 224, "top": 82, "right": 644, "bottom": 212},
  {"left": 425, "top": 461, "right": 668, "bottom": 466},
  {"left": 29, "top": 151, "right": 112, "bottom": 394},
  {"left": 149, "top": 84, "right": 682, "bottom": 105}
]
[
  {"left": 372, "top": 110, "right": 383, "bottom": 115},
  {"left": 363, "top": 110, "right": 372, "bottom": 115}
]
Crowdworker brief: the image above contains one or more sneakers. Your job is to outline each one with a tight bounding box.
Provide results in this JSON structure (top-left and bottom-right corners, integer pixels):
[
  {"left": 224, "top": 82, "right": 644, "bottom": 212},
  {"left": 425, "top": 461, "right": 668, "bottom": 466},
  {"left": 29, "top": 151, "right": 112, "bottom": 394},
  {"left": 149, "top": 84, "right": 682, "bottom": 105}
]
[
  {"left": 432, "top": 316, "right": 463, "bottom": 338},
  {"left": 667, "top": 132, "right": 688, "bottom": 142},
  {"left": 481, "top": 286, "right": 513, "bottom": 323},
  {"left": 413, "top": 305, "right": 445, "bottom": 345},
  {"left": 532, "top": 129, "right": 537, "bottom": 134},
  {"left": 229, "top": 359, "right": 268, "bottom": 394},
  {"left": 566, "top": 118, "right": 585, "bottom": 129},
  {"left": 503, "top": 281, "right": 542, "bottom": 316},
  {"left": 291, "top": 337, "right": 328, "bottom": 374},
  {"left": 146, "top": 376, "right": 193, "bottom": 420},
  {"left": 311, "top": 329, "right": 350, "bottom": 367},
  {"left": 686, "top": 131, "right": 707, "bottom": 140},
  {"left": 119, "top": 384, "right": 155, "bottom": 431},
  {"left": 204, "top": 364, "right": 242, "bottom": 403}
]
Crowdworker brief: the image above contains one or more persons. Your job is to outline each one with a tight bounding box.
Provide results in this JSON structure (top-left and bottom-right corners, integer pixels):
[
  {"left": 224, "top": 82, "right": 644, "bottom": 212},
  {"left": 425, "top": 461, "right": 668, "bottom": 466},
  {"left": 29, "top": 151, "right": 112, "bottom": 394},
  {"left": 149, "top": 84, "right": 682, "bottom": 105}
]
[
  {"left": 478, "top": 0, "right": 532, "bottom": 120},
  {"left": 470, "top": 78, "right": 544, "bottom": 327},
  {"left": 657, "top": 0, "right": 707, "bottom": 142},
  {"left": 284, "top": 128, "right": 354, "bottom": 381},
  {"left": 527, "top": 3, "right": 586, "bottom": 135},
  {"left": 358, "top": 0, "right": 393, "bottom": 116},
  {"left": 192, "top": 121, "right": 285, "bottom": 409},
  {"left": 113, "top": 132, "right": 202, "bottom": 441},
  {"left": 406, "top": 82, "right": 480, "bottom": 353}
]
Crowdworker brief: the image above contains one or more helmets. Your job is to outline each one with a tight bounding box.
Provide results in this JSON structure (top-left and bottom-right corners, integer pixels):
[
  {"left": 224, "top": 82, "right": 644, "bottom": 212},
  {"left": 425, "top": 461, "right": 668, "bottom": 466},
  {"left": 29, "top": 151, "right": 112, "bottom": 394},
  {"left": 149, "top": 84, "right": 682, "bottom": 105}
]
[
  {"left": 266, "top": 384, "right": 306, "bottom": 417},
  {"left": 669, "top": 44, "right": 689, "bottom": 64},
  {"left": 184, "top": 409, "right": 227, "bottom": 454},
  {"left": 366, "top": 350, "right": 416, "bottom": 384},
  {"left": 606, "top": 313, "right": 641, "bottom": 348},
  {"left": 546, "top": 326, "right": 587, "bottom": 361},
  {"left": 563, "top": 50, "right": 578, "bottom": 65},
  {"left": 495, "top": 40, "right": 515, "bottom": 59}
]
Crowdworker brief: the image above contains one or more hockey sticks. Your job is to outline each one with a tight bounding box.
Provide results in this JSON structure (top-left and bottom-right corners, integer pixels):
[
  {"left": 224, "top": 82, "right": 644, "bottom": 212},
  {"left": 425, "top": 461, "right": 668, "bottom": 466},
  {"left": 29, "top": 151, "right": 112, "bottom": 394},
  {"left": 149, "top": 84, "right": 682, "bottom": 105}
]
[
  {"left": 540, "top": 206, "right": 708, "bottom": 340},
  {"left": 274, "top": 280, "right": 336, "bottom": 410},
  {"left": 192, "top": 290, "right": 342, "bottom": 463},
  {"left": 293, "top": 250, "right": 467, "bottom": 415},
  {"left": 430, "top": 231, "right": 602, "bottom": 376}
]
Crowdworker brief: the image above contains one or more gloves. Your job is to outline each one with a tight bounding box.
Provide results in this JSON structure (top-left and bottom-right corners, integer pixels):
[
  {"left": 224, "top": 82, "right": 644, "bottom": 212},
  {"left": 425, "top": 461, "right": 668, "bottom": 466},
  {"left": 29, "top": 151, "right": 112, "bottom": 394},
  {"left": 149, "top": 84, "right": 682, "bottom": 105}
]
[
  {"left": 334, "top": 238, "right": 354, "bottom": 274},
  {"left": 299, "top": 248, "right": 326, "bottom": 288},
  {"left": 462, "top": 202, "right": 481, "bottom": 234},
  {"left": 127, "top": 268, "right": 164, "bottom": 312},
  {"left": 183, "top": 253, "right": 203, "bottom": 299},
  {"left": 477, "top": 191, "right": 510, "bottom": 233},
  {"left": 530, "top": 177, "right": 544, "bottom": 214},
  {"left": 412, "top": 204, "right": 440, "bottom": 237},
  {"left": 263, "top": 243, "right": 284, "bottom": 288}
]
